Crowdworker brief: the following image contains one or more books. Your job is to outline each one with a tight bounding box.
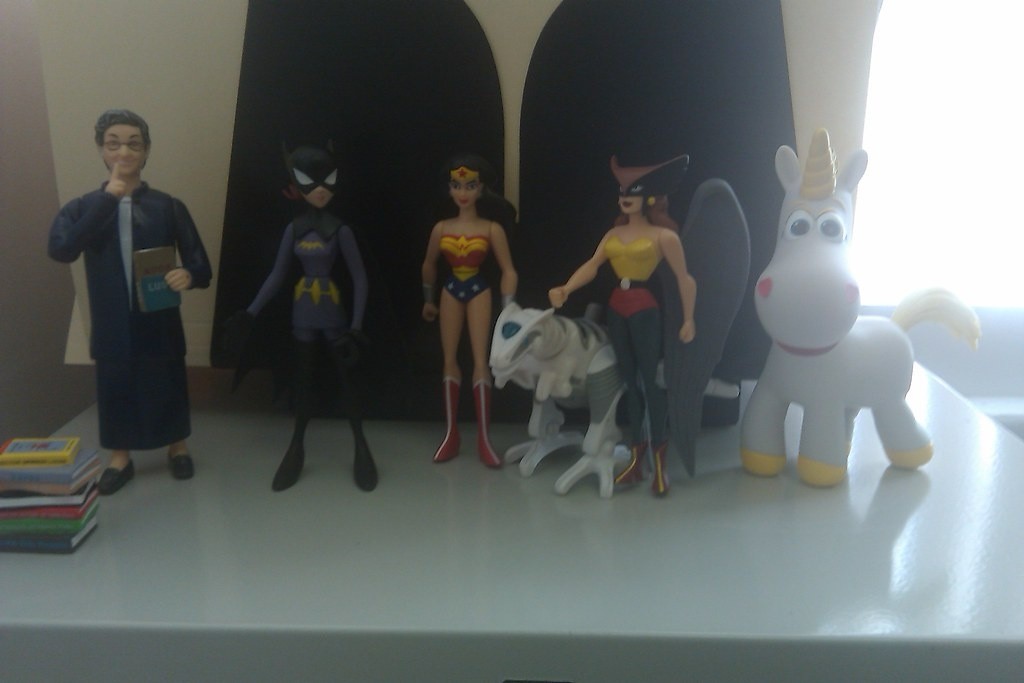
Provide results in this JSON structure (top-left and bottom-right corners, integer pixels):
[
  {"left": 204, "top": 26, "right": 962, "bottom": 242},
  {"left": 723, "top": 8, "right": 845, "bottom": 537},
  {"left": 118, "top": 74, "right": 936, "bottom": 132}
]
[{"left": 0, "top": 437, "right": 102, "bottom": 552}]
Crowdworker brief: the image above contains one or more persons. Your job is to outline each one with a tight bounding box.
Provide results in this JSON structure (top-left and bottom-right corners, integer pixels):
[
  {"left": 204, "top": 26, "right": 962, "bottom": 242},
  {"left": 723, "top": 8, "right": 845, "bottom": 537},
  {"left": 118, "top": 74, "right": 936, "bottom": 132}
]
[
  {"left": 421, "top": 147, "right": 519, "bottom": 468},
  {"left": 548, "top": 154, "right": 696, "bottom": 496},
  {"left": 214, "top": 146, "right": 380, "bottom": 492},
  {"left": 47, "top": 108, "right": 212, "bottom": 496}
]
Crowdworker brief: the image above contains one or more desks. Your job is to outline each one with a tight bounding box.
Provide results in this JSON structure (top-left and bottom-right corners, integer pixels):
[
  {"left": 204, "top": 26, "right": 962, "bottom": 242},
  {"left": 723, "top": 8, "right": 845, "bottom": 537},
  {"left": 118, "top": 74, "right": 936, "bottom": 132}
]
[{"left": 1, "top": 362, "right": 1024, "bottom": 683}]
